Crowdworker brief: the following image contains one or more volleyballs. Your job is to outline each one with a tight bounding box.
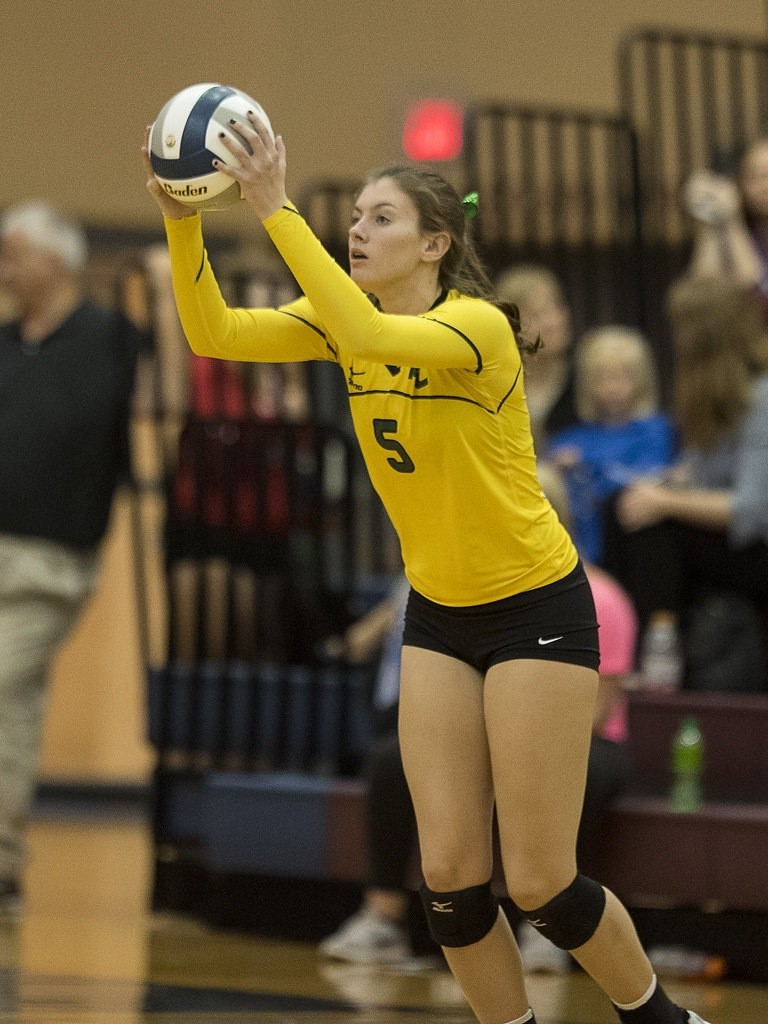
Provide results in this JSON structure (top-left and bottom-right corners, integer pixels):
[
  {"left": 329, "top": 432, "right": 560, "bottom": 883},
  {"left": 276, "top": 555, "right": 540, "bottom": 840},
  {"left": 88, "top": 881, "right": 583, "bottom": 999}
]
[{"left": 147, "top": 82, "right": 276, "bottom": 212}]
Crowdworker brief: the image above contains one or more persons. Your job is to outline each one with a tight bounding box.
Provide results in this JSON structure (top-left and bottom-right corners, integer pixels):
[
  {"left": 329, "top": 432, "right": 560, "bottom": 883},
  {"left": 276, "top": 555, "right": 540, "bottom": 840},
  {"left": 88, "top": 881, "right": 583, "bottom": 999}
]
[
  {"left": 140, "top": 109, "right": 710, "bottom": 1024},
  {"left": 0, "top": 202, "right": 154, "bottom": 901},
  {"left": 167, "top": 141, "right": 768, "bottom": 977}
]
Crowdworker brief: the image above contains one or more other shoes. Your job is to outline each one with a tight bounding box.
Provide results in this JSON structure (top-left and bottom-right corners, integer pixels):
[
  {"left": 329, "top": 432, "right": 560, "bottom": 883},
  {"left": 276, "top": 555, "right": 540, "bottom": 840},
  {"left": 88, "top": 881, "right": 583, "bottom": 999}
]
[
  {"left": 519, "top": 923, "right": 567, "bottom": 978},
  {"left": 687, "top": 1010, "right": 711, "bottom": 1024},
  {"left": 319, "top": 911, "right": 407, "bottom": 962}
]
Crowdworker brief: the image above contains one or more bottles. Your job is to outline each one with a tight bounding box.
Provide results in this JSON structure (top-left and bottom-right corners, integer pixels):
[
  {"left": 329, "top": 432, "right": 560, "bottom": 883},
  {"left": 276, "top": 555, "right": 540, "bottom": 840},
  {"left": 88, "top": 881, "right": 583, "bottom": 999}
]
[
  {"left": 644, "top": 610, "right": 682, "bottom": 687},
  {"left": 648, "top": 944, "right": 724, "bottom": 982},
  {"left": 671, "top": 718, "right": 705, "bottom": 815}
]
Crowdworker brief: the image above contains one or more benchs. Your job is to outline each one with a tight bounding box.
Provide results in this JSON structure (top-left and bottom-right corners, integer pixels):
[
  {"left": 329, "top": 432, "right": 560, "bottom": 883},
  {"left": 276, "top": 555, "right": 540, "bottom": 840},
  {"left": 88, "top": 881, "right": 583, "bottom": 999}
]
[{"left": 146, "top": 570, "right": 768, "bottom": 911}]
